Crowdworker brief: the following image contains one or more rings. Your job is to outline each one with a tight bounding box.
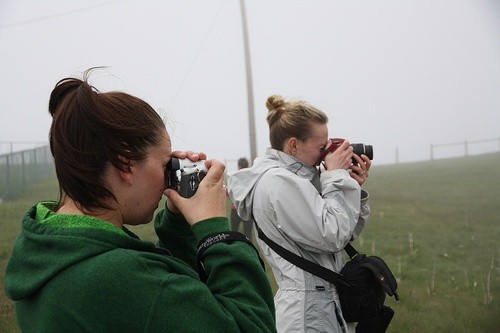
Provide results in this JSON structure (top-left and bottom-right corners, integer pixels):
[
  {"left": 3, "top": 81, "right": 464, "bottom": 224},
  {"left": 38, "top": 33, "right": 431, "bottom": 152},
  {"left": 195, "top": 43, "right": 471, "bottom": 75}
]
[
  {"left": 223, "top": 183, "right": 226, "bottom": 188},
  {"left": 360, "top": 171, "right": 365, "bottom": 176}
]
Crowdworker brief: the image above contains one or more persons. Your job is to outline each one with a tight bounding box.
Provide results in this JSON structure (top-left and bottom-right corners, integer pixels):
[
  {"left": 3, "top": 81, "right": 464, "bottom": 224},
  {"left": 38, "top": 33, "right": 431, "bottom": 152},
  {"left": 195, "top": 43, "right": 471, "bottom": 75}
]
[
  {"left": 228, "top": 159, "right": 253, "bottom": 240},
  {"left": 226, "top": 95, "right": 371, "bottom": 333},
  {"left": 4, "top": 66, "right": 278, "bottom": 333}
]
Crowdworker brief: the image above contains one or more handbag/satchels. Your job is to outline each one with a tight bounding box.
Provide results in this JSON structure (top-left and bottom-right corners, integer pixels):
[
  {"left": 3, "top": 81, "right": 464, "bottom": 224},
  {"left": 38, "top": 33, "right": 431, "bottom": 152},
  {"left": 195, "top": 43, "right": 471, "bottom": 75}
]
[{"left": 336, "top": 253, "right": 400, "bottom": 325}]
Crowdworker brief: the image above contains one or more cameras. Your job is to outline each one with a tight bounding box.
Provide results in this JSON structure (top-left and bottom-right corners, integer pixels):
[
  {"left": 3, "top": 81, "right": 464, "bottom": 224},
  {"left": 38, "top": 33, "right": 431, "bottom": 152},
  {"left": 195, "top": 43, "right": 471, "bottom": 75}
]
[
  {"left": 167, "top": 156, "right": 209, "bottom": 199},
  {"left": 321, "top": 138, "right": 374, "bottom": 165}
]
